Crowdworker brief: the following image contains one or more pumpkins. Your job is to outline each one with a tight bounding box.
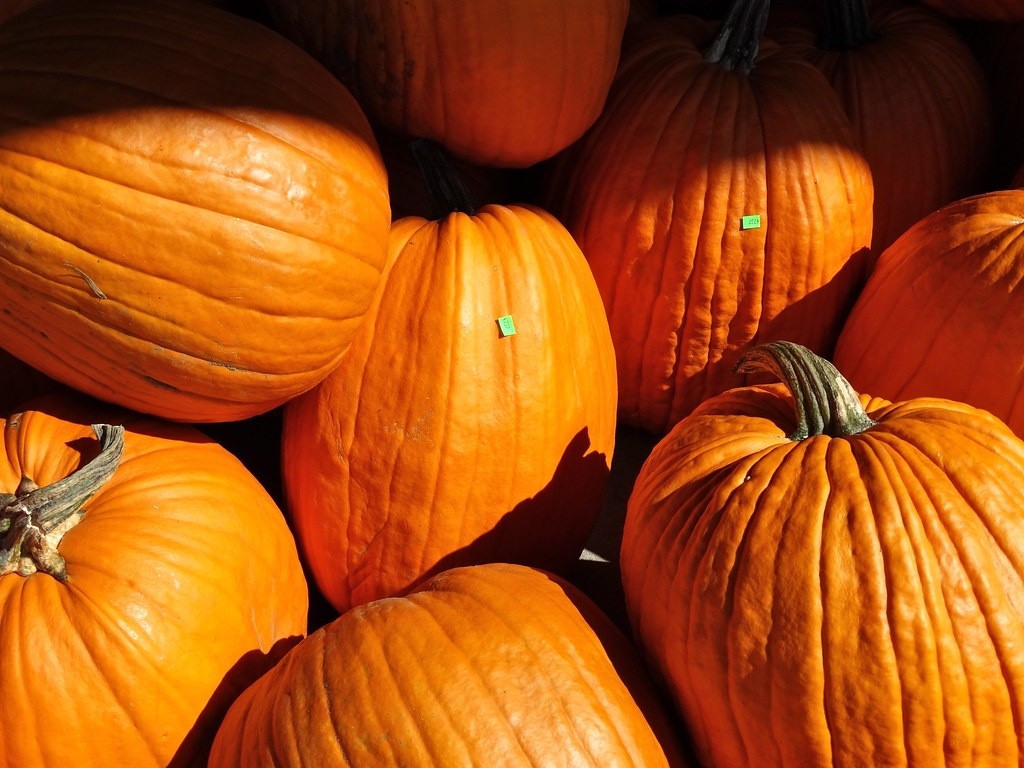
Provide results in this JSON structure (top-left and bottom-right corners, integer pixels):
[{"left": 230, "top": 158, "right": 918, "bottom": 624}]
[{"left": 0, "top": 0, "right": 1024, "bottom": 768}]
